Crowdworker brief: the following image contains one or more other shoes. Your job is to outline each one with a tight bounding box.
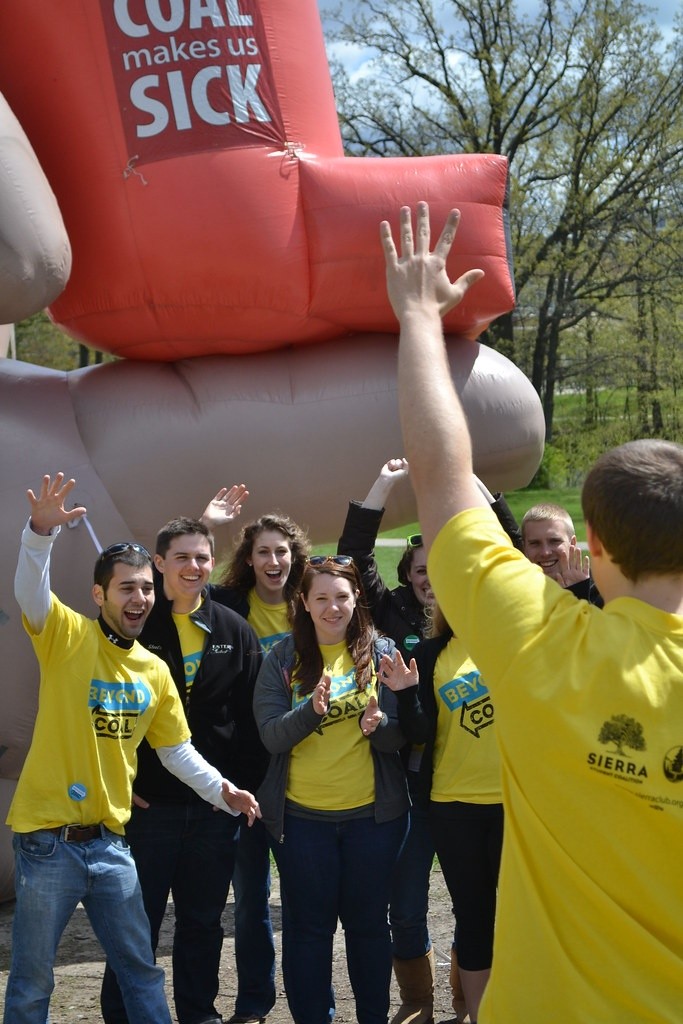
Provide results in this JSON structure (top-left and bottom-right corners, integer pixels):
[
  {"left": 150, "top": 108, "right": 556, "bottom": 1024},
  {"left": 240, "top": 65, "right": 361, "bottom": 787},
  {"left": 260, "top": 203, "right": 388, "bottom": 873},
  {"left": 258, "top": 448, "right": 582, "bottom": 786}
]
[
  {"left": 225, "top": 1015, "right": 266, "bottom": 1024},
  {"left": 197, "top": 1015, "right": 223, "bottom": 1024}
]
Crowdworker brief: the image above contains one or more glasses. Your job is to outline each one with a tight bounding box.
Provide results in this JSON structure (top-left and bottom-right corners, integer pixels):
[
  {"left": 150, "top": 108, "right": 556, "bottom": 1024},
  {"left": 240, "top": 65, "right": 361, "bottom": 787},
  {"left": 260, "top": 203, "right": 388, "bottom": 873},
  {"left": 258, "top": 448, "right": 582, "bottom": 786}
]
[
  {"left": 406, "top": 534, "right": 423, "bottom": 551},
  {"left": 101, "top": 543, "right": 152, "bottom": 564},
  {"left": 303, "top": 556, "right": 357, "bottom": 574}
]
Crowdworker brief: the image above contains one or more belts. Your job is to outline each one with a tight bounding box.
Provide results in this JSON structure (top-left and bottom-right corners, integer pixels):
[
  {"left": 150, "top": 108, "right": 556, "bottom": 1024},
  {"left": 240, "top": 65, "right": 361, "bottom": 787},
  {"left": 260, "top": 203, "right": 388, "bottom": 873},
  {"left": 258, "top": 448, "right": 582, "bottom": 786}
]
[{"left": 39, "top": 824, "right": 108, "bottom": 843}]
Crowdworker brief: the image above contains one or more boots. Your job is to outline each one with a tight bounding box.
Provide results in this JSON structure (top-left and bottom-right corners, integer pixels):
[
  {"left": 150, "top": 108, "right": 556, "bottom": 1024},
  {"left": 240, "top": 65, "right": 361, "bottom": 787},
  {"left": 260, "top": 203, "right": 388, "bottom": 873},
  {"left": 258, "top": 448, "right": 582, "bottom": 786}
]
[
  {"left": 449, "top": 943, "right": 471, "bottom": 1024},
  {"left": 390, "top": 943, "right": 436, "bottom": 1024}
]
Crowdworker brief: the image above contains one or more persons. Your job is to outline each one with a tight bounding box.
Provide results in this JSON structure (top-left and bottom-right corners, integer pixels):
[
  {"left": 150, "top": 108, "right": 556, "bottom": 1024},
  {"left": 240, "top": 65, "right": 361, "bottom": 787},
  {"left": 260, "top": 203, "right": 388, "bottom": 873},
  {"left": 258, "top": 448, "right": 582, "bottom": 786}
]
[
  {"left": 333, "top": 455, "right": 523, "bottom": 1024},
  {"left": 5, "top": 471, "right": 263, "bottom": 1024},
  {"left": 254, "top": 558, "right": 404, "bottom": 1024},
  {"left": 196, "top": 484, "right": 312, "bottom": 1024},
  {"left": 382, "top": 197, "right": 683, "bottom": 1024},
  {"left": 99, "top": 515, "right": 264, "bottom": 1024},
  {"left": 519, "top": 503, "right": 605, "bottom": 611}
]
